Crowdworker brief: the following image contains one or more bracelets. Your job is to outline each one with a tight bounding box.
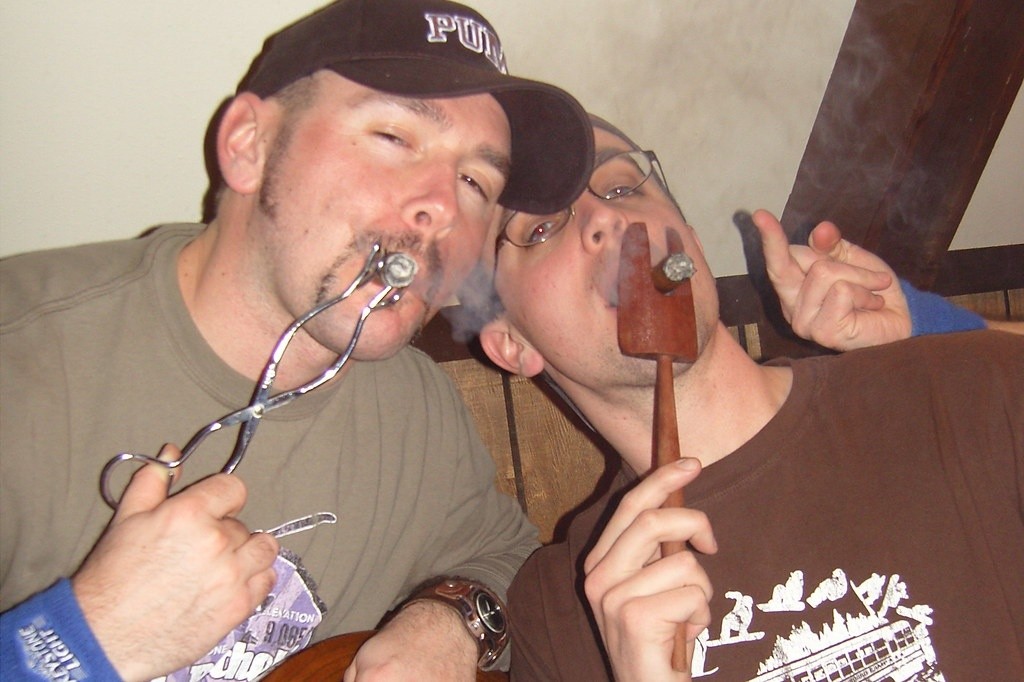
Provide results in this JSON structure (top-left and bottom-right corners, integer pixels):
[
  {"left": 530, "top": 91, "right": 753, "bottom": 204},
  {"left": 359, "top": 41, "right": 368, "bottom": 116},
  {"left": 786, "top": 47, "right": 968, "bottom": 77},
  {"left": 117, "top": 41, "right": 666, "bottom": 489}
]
[
  {"left": 902, "top": 282, "right": 987, "bottom": 349},
  {"left": 1, "top": 574, "right": 126, "bottom": 680}
]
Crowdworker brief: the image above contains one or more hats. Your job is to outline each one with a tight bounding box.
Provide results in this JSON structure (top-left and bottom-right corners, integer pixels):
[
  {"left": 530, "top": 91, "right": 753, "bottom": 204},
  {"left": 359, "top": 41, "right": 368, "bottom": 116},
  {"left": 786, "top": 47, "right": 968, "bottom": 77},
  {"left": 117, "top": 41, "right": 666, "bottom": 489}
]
[{"left": 238, "top": 0, "right": 596, "bottom": 213}]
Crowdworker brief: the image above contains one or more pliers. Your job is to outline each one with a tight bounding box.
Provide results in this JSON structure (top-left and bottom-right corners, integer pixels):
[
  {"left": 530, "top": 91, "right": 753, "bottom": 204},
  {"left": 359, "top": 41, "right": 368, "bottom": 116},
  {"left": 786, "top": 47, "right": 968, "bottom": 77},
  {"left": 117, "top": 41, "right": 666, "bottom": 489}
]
[{"left": 98, "top": 236, "right": 408, "bottom": 510}]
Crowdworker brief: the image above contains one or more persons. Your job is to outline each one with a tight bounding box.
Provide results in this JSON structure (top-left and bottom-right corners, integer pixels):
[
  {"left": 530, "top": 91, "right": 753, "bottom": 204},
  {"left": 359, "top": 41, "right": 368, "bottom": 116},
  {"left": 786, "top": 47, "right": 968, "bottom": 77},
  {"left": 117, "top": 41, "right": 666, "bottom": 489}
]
[
  {"left": 750, "top": 210, "right": 1023, "bottom": 352},
  {"left": 0, "top": 0, "right": 594, "bottom": 682},
  {"left": 480, "top": 113, "right": 1023, "bottom": 682}
]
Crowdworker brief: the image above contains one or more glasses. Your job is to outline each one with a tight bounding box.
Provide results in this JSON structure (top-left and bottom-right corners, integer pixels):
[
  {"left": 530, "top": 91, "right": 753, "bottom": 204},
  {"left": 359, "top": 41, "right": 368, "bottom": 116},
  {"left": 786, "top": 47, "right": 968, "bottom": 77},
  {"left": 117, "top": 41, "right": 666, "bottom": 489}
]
[{"left": 493, "top": 149, "right": 687, "bottom": 282}]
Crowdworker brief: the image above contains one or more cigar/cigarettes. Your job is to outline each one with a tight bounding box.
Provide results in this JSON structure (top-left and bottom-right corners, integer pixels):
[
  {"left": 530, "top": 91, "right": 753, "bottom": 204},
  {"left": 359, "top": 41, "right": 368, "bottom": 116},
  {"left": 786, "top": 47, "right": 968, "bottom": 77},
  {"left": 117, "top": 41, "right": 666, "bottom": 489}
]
[
  {"left": 378, "top": 253, "right": 418, "bottom": 288},
  {"left": 653, "top": 253, "right": 696, "bottom": 294}
]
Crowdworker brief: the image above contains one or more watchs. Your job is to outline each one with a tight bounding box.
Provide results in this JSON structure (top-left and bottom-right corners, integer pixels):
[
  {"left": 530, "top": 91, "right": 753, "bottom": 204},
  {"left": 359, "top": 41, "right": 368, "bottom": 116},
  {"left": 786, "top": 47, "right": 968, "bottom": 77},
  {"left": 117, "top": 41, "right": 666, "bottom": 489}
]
[{"left": 373, "top": 576, "right": 509, "bottom": 667}]
